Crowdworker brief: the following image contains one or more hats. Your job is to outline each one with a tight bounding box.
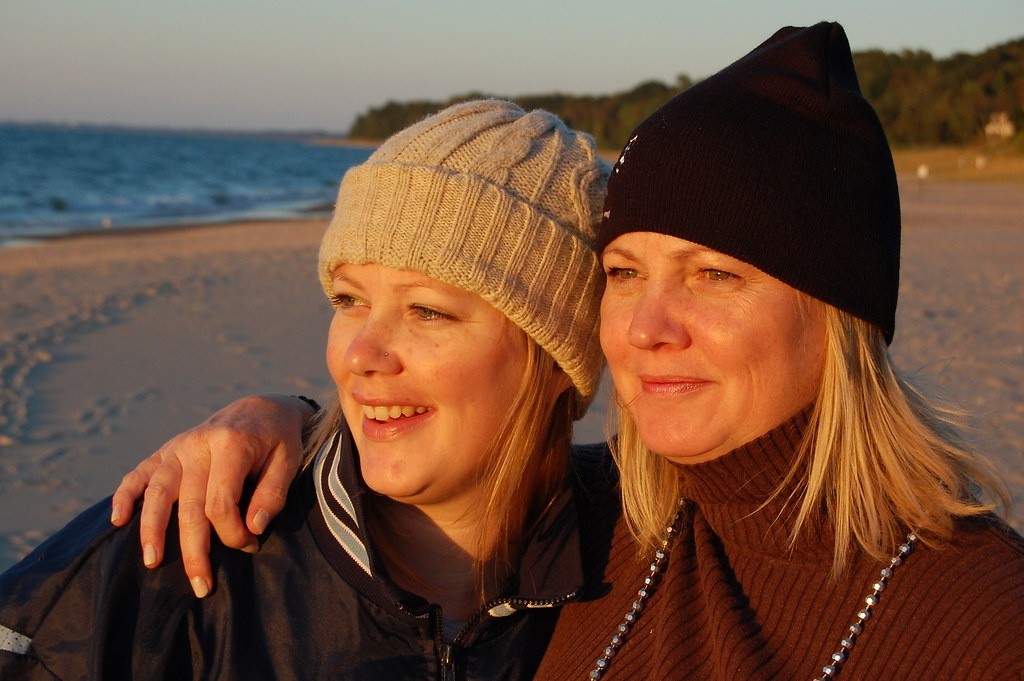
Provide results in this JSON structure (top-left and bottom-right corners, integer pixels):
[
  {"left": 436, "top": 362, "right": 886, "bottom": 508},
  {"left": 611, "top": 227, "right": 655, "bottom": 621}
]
[
  {"left": 595, "top": 21, "right": 902, "bottom": 347},
  {"left": 318, "top": 90, "right": 617, "bottom": 422}
]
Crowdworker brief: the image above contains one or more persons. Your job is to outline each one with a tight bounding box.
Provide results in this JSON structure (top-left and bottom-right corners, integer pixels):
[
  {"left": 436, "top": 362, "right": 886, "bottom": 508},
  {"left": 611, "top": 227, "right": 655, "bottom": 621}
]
[
  {"left": 0, "top": 99, "right": 622, "bottom": 681},
  {"left": 110, "top": 18, "right": 1024, "bottom": 681}
]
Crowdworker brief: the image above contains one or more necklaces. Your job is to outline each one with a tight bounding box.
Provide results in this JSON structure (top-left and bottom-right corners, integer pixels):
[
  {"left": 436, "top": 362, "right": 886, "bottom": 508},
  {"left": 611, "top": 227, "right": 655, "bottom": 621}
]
[{"left": 589, "top": 476, "right": 946, "bottom": 681}]
